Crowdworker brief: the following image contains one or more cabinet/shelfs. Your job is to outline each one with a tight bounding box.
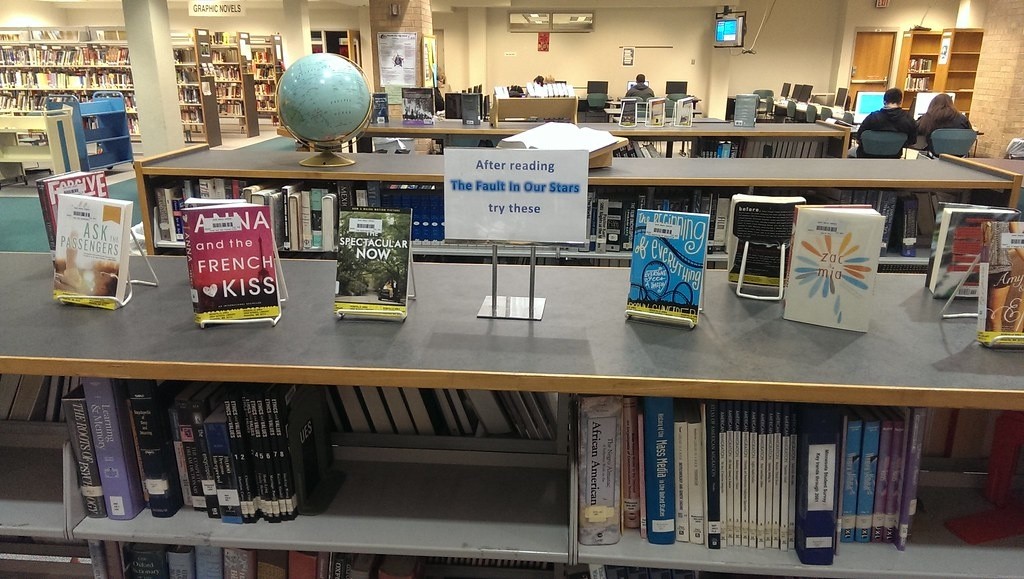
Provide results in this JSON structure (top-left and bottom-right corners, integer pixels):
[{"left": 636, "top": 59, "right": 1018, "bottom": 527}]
[
  {"left": 0, "top": 26, "right": 285, "bottom": 190},
  {"left": 275, "top": 119, "right": 850, "bottom": 155},
  {"left": 132, "top": 145, "right": 1023, "bottom": 270},
  {"left": 0, "top": 250, "right": 1024, "bottom": 579},
  {"left": 895, "top": 29, "right": 983, "bottom": 116}
]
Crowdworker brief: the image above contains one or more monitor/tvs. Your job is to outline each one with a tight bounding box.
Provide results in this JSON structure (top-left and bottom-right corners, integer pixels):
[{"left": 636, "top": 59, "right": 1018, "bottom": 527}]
[
  {"left": 792, "top": 84, "right": 813, "bottom": 102},
  {"left": 781, "top": 83, "right": 791, "bottom": 101},
  {"left": 587, "top": 81, "right": 608, "bottom": 94},
  {"left": 714, "top": 16, "right": 744, "bottom": 48},
  {"left": 854, "top": 92, "right": 886, "bottom": 124},
  {"left": 913, "top": 92, "right": 955, "bottom": 121},
  {"left": 835, "top": 88, "right": 849, "bottom": 107},
  {"left": 627, "top": 81, "right": 649, "bottom": 91},
  {"left": 666, "top": 81, "right": 688, "bottom": 94}
]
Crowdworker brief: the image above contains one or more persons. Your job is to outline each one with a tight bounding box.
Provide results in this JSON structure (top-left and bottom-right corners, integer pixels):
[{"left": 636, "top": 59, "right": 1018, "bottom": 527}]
[
  {"left": 534, "top": 76, "right": 544, "bottom": 87},
  {"left": 916, "top": 93, "right": 975, "bottom": 159},
  {"left": 856, "top": 88, "right": 918, "bottom": 159},
  {"left": 625, "top": 74, "right": 654, "bottom": 107}
]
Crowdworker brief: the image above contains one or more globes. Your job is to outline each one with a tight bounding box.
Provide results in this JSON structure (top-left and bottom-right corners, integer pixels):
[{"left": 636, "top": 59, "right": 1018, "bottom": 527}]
[{"left": 274, "top": 52, "right": 374, "bottom": 167}]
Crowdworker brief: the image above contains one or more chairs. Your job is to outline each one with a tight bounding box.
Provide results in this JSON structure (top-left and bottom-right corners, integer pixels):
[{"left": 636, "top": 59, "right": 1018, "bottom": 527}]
[{"left": 586, "top": 90, "right": 978, "bottom": 160}]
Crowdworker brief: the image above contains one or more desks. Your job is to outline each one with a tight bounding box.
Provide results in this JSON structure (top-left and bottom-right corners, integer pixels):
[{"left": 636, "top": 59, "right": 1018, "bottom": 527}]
[
  {"left": 760, "top": 99, "right": 985, "bottom": 137},
  {"left": 520, "top": 95, "right": 587, "bottom": 102},
  {"left": 606, "top": 101, "right": 621, "bottom": 108},
  {"left": 604, "top": 107, "right": 702, "bottom": 156}
]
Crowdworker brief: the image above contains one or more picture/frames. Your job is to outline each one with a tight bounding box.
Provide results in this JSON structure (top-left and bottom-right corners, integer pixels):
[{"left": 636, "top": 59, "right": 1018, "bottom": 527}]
[{"left": 421, "top": 35, "right": 438, "bottom": 89}]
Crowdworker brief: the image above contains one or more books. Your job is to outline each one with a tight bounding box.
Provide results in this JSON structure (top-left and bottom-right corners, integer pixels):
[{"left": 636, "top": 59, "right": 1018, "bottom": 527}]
[
  {"left": 493, "top": 82, "right": 575, "bottom": 120},
  {"left": 621, "top": 99, "right": 637, "bottom": 124},
  {"left": 0, "top": 31, "right": 283, "bottom": 146},
  {"left": 372, "top": 93, "right": 388, "bottom": 123},
  {"left": 649, "top": 98, "right": 665, "bottom": 125},
  {"left": 905, "top": 58, "right": 932, "bottom": 90},
  {"left": 675, "top": 98, "right": 694, "bottom": 127},
  {"left": 36, "top": 138, "right": 1024, "bottom": 351},
  {"left": 0, "top": 374, "right": 1024, "bottom": 579}
]
